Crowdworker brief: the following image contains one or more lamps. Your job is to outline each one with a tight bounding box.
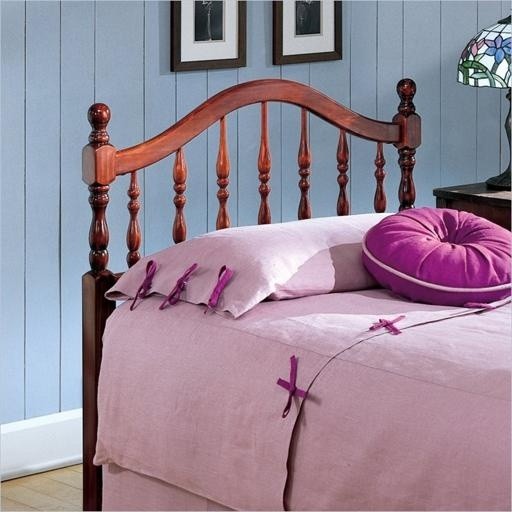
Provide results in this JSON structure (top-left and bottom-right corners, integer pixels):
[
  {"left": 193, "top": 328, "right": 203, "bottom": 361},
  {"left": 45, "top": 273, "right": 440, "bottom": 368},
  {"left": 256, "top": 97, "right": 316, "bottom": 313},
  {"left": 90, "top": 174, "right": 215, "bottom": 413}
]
[{"left": 455, "top": 15, "right": 512, "bottom": 191}]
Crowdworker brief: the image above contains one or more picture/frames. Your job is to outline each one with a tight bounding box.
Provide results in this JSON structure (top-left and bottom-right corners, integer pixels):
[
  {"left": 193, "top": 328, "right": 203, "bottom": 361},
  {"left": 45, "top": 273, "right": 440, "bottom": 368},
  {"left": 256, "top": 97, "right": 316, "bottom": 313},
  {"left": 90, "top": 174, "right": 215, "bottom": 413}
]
[{"left": 170, "top": 1, "right": 343, "bottom": 73}]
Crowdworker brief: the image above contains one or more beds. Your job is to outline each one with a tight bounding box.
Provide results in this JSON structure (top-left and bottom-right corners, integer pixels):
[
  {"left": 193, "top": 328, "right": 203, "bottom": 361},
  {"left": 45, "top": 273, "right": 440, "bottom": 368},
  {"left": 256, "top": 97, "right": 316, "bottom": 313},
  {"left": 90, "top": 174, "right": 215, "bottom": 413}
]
[{"left": 80, "top": 80, "right": 512, "bottom": 511}]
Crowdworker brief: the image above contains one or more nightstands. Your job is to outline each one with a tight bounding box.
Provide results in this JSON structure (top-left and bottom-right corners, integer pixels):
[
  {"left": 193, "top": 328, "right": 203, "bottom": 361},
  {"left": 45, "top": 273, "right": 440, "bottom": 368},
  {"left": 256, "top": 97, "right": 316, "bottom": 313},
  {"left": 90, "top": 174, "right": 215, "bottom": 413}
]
[{"left": 432, "top": 183, "right": 512, "bottom": 229}]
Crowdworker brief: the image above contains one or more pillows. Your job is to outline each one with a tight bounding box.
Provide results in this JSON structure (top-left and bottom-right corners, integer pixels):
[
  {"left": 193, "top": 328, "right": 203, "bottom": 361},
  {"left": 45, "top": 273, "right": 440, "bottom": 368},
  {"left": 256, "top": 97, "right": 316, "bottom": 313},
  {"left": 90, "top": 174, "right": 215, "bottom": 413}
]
[
  {"left": 362, "top": 207, "right": 511, "bottom": 310},
  {"left": 104, "top": 212, "right": 398, "bottom": 316}
]
